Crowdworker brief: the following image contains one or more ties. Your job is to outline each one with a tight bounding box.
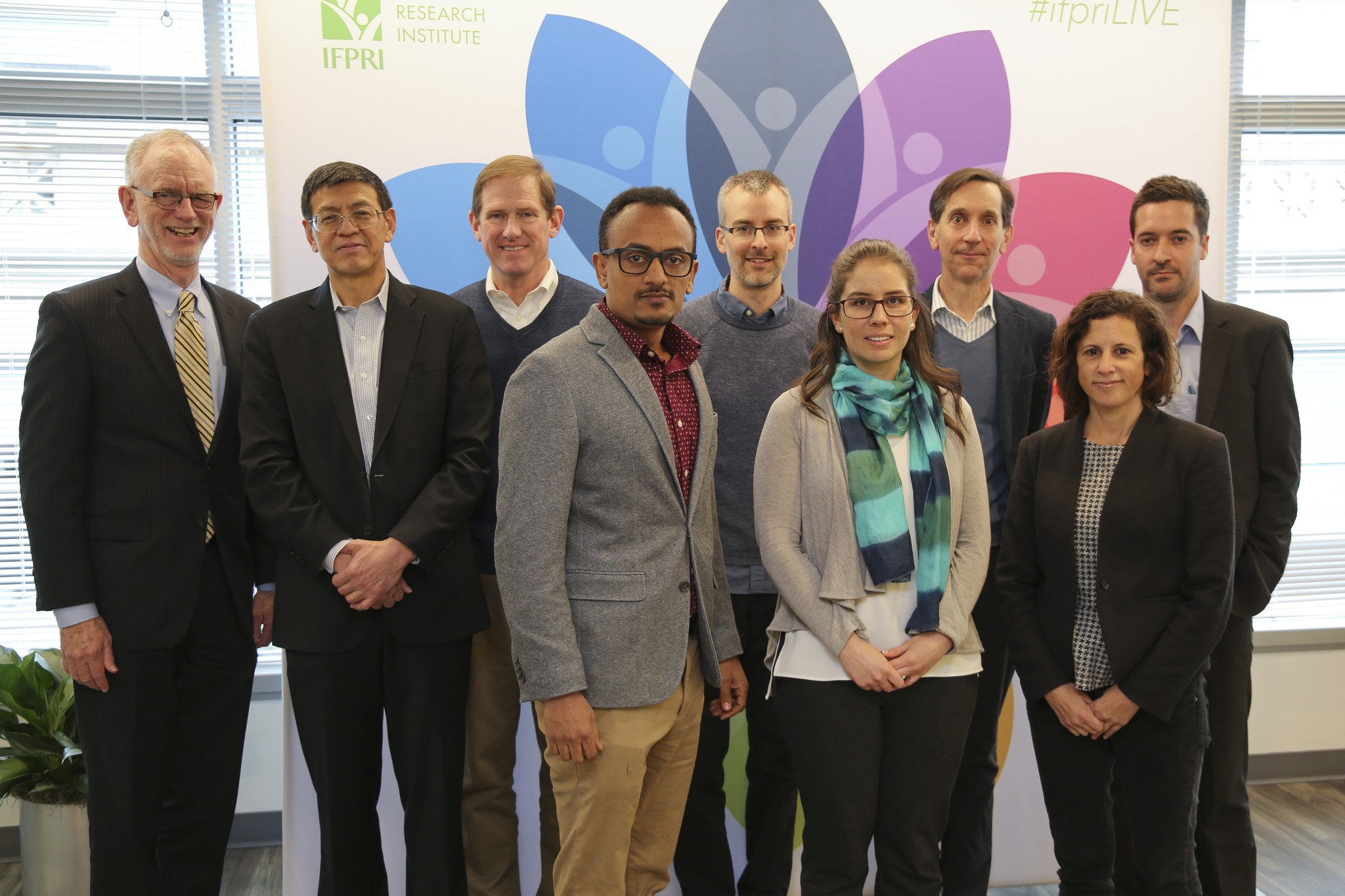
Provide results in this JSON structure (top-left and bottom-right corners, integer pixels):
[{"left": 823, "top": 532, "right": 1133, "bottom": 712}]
[{"left": 174, "top": 289, "right": 215, "bottom": 543}]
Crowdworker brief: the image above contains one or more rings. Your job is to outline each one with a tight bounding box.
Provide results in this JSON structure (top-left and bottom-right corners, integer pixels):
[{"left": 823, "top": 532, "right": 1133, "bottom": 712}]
[{"left": 902, "top": 675, "right": 906, "bottom": 679}]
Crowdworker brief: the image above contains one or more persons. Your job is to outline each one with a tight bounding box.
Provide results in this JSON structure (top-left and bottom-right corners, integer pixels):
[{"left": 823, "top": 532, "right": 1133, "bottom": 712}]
[
  {"left": 1128, "top": 175, "right": 1300, "bottom": 896},
  {"left": 237, "top": 160, "right": 489, "bottom": 896},
  {"left": 669, "top": 168, "right": 826, "bottom": 896},
  {"left": 494, "top": 189, "right": 750, "bottom": 896},
  {"left": 913, "top": 168, "right": 1058, "bottom": 896},
  {"left": 17, "top": 128, "right": 278, "bottom": 896},
  {"left": 992, "top": 290, "right": 1236, "bottom": 896},
  {"left": 446, "top": 156, "right": 605, "bottom": 896},
  {"left": 752, "top": 238, "right": 993, "bottom": 896}
]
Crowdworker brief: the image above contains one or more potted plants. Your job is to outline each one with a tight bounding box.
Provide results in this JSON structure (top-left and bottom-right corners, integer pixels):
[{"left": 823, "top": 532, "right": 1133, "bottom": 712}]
[{"left": 0, "top": 644, "right": 92, "bottom": 896}]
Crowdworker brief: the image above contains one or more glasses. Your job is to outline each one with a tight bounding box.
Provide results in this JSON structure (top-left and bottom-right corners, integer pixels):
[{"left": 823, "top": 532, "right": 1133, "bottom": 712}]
[
  {"left": 307, "top": 207, "right": 387, "bottom": 235},
  {"left": 834, "top": 296, "right": 917, "bottom": 319},
  {"left": 720, "top": 225, "right": 790, "bottom": 239},
  {"left": 602, "top": 248, "right": 697, "bottom": 278},
  {"left": 132, "top": 186, "right": 219, "bottom": 212}
]
